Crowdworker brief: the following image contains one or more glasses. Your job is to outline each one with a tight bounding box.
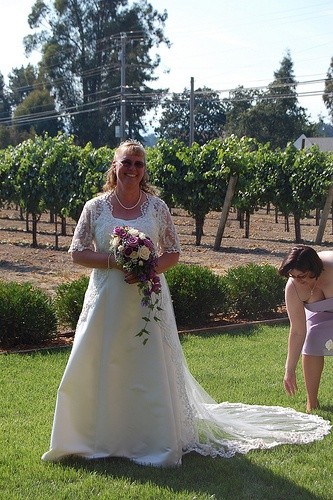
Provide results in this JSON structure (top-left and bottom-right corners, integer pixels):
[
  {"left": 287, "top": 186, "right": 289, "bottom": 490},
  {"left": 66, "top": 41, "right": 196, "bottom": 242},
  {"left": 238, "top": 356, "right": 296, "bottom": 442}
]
[
  {"left": 287, "top": 272, "right": 309, "bottom": 279},
  {"left": 117, "top": 159, "right": 145, "bottom": 168}
]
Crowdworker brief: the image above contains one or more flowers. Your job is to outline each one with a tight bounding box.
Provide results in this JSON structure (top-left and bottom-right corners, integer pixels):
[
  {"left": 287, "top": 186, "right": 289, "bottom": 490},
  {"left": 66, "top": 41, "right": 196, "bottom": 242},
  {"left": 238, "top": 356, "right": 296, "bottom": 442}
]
[{"left": 106, "top": 223, "right": 165, "bottom": 346}]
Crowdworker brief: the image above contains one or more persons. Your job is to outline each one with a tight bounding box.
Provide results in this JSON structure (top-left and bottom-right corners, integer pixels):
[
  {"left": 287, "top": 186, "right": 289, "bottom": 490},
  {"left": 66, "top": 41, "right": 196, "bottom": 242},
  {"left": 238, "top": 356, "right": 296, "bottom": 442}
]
[
  {"left": 41, "top": 140, "right": 332, "bottom": 469},
  {"left": 278, "top": 245, "right": 333, "bottom": 415}
]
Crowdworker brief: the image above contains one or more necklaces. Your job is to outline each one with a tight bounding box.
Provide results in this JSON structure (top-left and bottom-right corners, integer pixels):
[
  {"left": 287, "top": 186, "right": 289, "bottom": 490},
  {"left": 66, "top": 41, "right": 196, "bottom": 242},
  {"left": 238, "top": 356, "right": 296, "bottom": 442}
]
[
  {"left": 311, "top": 277, "right": 319, "bottom": 295},
  {"left": 113, "top": 191, "right": 142, "bottom": 209}
]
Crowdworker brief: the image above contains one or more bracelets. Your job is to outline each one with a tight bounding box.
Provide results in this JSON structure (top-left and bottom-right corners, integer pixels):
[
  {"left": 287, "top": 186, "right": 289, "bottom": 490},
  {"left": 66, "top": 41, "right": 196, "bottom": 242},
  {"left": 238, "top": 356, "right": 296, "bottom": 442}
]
[{"left": 108, "top": 255, "right": 109, "bottom": 269}]
